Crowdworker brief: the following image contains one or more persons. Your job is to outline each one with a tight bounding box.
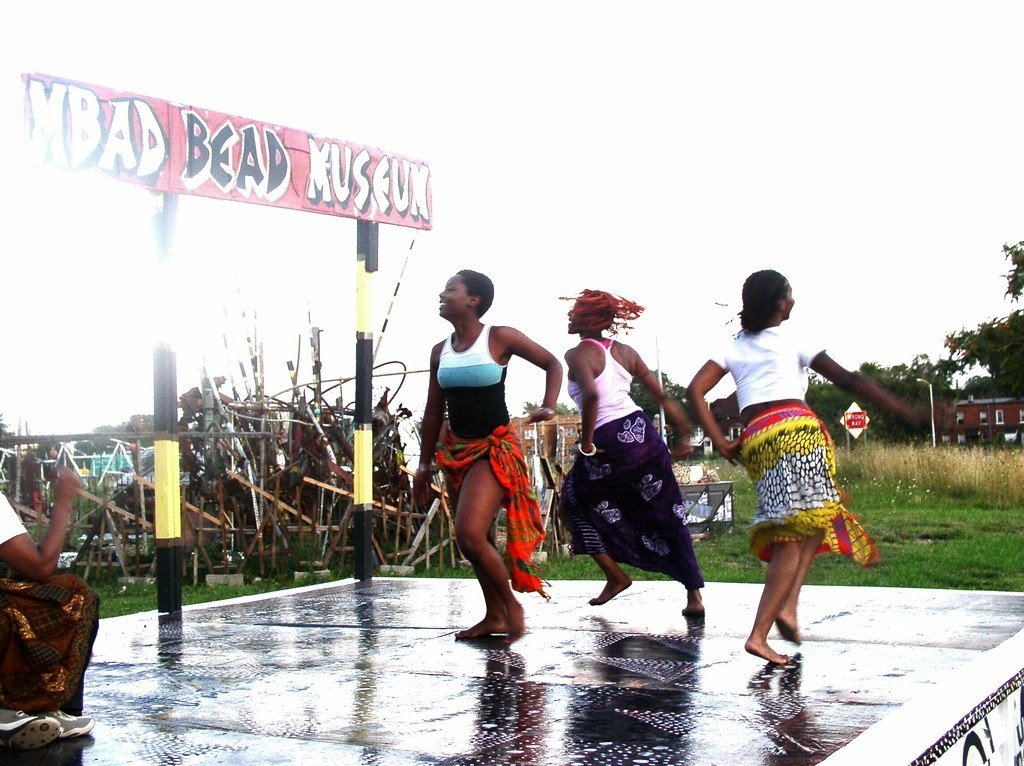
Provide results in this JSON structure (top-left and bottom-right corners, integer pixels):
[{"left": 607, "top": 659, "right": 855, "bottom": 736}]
[
  {"left": 559, "top": 288, "right": 705, "bottom": 615},
  {"left": 0, "top": 469, "right": 101, "bottom": 749},
  {"left": 412, "top": 269, "right": 564, "bottom": 636},
  {"left": 686, "top": 270, "right": 910, "bottom": 664}
]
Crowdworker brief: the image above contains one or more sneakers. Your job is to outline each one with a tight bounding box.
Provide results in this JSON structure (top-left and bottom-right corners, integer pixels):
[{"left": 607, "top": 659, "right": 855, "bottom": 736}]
[
  {"left": 31, "top": 708, "right": 95, "bottom": 737},
  {"left": 0, "top": 705, "right": 61, "bottom": 748}
]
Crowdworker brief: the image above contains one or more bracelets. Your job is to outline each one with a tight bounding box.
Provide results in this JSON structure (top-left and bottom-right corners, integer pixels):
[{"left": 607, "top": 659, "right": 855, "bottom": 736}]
[
  {"left": 419, "top": 464, "right": 431, "bottom": 471},
  {"left": 540, "top": 407, "right": 556, "bottom": 417},
  {"left": 577, "top": 443, "right": 597, "bottom": 457}
]
